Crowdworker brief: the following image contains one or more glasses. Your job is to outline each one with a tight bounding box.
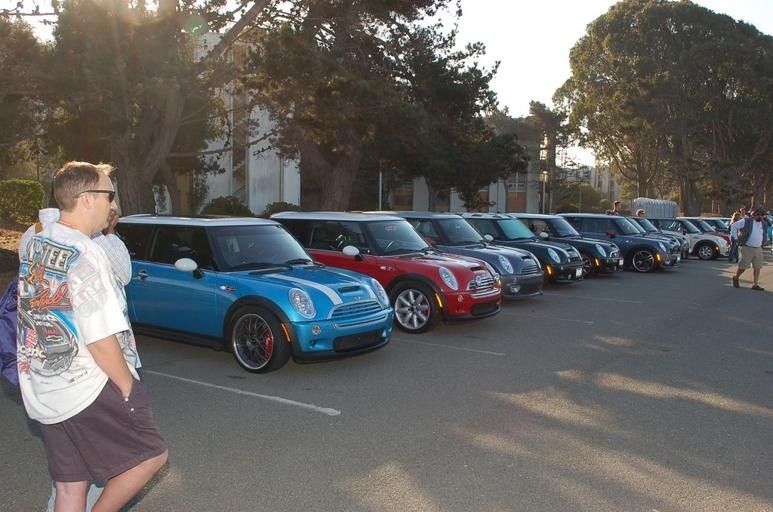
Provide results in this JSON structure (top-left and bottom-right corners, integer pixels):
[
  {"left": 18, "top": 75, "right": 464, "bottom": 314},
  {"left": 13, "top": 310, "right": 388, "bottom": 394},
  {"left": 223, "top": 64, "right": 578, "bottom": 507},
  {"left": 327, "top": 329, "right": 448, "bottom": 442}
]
[{"left": 73, "top": 189, "right": 116, "bottom": 203}]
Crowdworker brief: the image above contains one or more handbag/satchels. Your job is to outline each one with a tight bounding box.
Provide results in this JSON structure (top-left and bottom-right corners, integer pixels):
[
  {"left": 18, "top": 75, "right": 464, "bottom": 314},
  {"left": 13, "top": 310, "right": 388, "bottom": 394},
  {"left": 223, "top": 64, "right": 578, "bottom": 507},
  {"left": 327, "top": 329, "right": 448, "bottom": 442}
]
[{"left": 0, "top": 277, "right": 19, "bottom": 388}]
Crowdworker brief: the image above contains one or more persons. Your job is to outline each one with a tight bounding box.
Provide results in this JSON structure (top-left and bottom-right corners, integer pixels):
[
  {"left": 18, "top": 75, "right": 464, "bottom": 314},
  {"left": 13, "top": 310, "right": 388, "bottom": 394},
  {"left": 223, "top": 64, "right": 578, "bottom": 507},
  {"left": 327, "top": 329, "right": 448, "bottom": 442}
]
[
  {"left": 729, "top": 211, "right": 744, "bottom": 263},
  {"left": 608, "top": 201, "right": 622, "bottom": 215},
  {"left": 740, "top": 207, "right": 749, "bottom": 218},
  {"left": 17, "top": 163, "right": 143, "bottom": 512},
  {"left": 636, "top": 209, "right": 645, "bottom": 217},
  {"left": 16, "top": 160, "right": 169, "bottom": 512},
  {"left": 731, "top": 207, "right": 773, "bottom": 290}
]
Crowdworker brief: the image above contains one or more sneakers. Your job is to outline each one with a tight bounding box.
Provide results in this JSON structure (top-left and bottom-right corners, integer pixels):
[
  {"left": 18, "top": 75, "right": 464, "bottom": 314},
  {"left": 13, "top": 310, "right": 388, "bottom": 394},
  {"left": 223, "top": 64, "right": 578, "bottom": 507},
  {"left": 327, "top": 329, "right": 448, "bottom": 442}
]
[
  {"left": 733, "top": 276, "right": 740, "bottom": 288},
  {"left": 752, "top": 285, "right": 764, "bottom": 290}
]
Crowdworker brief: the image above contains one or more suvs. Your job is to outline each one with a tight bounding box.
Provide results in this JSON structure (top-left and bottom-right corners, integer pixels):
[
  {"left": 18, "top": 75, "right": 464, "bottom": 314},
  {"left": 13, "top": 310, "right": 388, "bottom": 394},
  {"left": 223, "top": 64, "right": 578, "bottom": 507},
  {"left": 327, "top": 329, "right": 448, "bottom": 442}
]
[
  {"left": 512, "top": 211, "right": 732, "bottom": 276},
  {"left": 118, "top": 211, "right": 395, "bottom": 374},
  {"left": 462, "top": 213, "right": 583, "bottom": 283},
  {"left": 265, "top": 209, "right": 503, "bottom": 335},
  {"left": 399, "top": 211, "right": 545, "bottom": 303}
]
